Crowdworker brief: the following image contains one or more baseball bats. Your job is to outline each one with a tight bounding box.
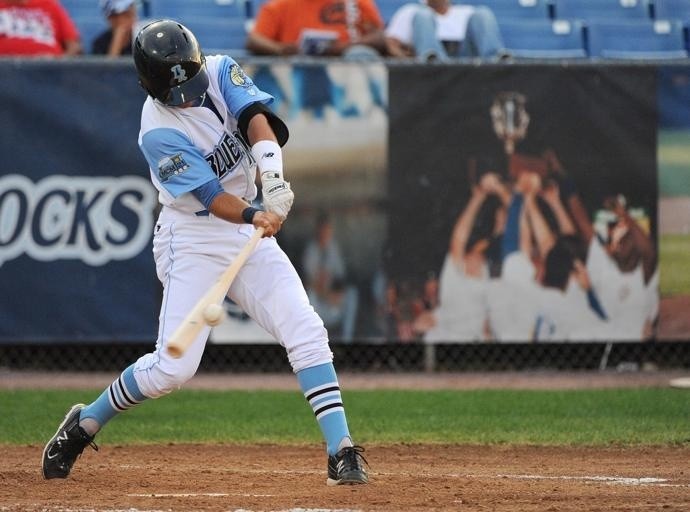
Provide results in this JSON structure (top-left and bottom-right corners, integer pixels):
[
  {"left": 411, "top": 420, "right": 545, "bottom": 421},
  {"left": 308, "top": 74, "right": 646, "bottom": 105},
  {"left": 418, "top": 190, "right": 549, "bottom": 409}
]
[{"left": 167, "top": 227, "right": 264, "bottom": 359}]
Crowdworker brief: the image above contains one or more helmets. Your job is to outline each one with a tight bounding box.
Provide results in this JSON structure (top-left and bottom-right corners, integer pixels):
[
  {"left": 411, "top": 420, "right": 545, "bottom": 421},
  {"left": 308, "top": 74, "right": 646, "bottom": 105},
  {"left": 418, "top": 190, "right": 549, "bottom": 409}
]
[{"left": 132, "top": 19, "right": 209, "bottom": 106}]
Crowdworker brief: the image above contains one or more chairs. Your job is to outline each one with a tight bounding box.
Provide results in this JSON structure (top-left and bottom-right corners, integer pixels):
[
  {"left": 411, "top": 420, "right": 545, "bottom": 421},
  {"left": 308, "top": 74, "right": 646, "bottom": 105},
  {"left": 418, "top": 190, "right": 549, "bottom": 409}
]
[
  {"left": 58, "top": 0, "right": 263, "bottom": 59},
  {"left": 494, "top": 0, "right": 690, "bottom": 62}
]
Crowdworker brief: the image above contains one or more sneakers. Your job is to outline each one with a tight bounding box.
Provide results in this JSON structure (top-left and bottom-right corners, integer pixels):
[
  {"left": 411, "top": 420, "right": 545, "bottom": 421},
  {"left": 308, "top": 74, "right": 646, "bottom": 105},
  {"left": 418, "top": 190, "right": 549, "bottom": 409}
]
[
  {"left": 41, "top": 403, "right": 98, "bottom": 479},
  {"left": 327, "top": 445, "right": 370, "bottom": 486}
]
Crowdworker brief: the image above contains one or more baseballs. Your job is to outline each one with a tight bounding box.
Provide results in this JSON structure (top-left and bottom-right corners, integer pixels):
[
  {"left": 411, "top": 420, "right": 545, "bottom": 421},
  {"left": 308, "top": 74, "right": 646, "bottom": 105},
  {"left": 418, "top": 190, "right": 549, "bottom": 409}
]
[{"left": 203, "top": 305, "right": 225, "bottom": 326}]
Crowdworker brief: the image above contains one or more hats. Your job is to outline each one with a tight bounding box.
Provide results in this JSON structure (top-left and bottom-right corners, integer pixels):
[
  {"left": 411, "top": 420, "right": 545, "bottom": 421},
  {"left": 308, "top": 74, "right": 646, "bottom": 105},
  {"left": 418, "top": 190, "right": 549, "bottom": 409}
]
[{"left": 100, "top": 0, "right": 135, "bottom": 17}]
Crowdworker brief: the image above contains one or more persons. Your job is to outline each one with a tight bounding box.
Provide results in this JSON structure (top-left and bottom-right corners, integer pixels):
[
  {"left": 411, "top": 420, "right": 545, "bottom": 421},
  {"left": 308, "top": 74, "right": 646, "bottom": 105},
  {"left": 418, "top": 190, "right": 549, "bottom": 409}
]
[
  {"left": 0, "top": 0, "right": 81, "bottom": 60},
  {"left": 385, "top": 0, "right": 513, "bottom": 63},
  {"left": 99, "top": 0, "right": 138, "bottom": 55},
  {"left": 247, "top": 0, "right": 384, "bottom": 59},
  {"left": 297, "top": 150, "right": 655, "bottom": 343},
  {"left": 41, "top": 21, "right": 366, "bottom": 487}
]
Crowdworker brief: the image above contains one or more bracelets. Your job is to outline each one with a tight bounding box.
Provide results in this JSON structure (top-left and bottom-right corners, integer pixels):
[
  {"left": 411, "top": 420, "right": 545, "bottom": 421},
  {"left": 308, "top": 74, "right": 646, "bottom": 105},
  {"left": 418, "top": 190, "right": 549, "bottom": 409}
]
[{"left": 242, "top": 208, "right": 262, "bottom": 224}]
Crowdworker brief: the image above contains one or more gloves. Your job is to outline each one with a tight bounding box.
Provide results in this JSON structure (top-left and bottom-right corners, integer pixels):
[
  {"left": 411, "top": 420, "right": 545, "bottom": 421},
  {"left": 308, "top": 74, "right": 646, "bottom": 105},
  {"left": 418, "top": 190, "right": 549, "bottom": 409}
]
[{"left": 260, "top": 170, "right": 294, "bottom": 222}]
